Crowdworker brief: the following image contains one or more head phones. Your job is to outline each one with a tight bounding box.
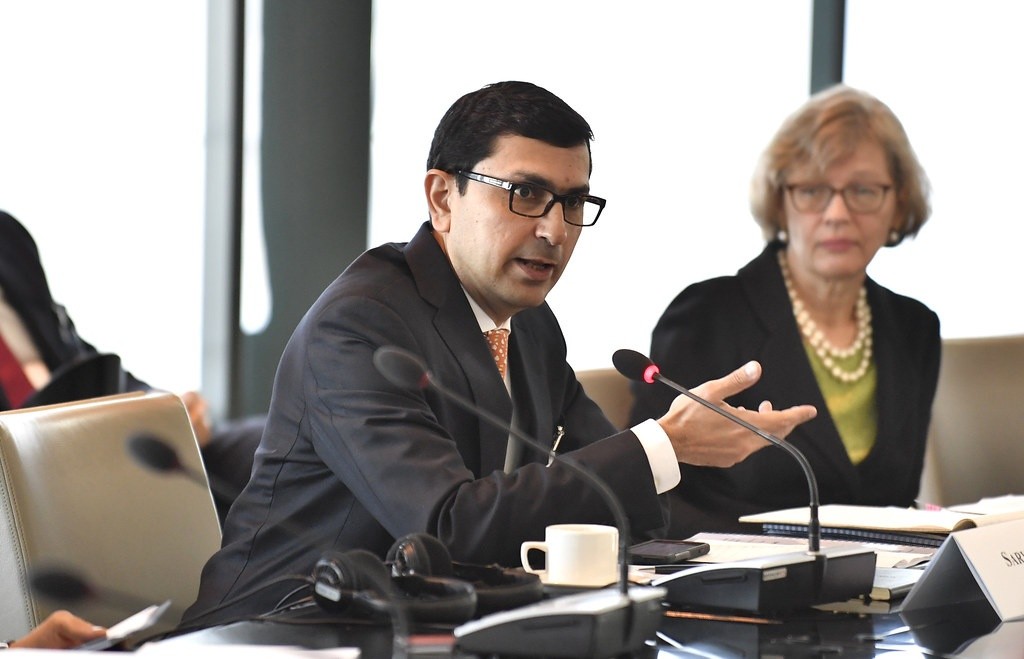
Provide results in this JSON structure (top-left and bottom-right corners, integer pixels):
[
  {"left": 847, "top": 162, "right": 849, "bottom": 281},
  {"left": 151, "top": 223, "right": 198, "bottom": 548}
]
[{"left": 308, "top": 534, "right": 543, "bottom": 623}]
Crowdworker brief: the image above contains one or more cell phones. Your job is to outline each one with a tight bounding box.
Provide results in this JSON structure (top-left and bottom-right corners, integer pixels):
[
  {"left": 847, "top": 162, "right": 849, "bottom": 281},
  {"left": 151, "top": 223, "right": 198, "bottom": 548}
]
[{"left": 626, "top": 537, "right": 710, "bottom": 568}]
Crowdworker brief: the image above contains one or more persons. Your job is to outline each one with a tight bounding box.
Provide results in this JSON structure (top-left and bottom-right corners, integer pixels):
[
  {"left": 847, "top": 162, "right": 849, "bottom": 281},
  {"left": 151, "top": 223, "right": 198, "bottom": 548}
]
[
  {"left": 169, "top": 81, "right": 815, "bottom": 637},
  {"left": 624, "top": 86, "right": 947, "bottom": 532},
  {"left": 0, "top": 210, "right": 273, "bottom": 532},
  {"left": 0, "top": 610, "right": 129, "bottom": 652}
]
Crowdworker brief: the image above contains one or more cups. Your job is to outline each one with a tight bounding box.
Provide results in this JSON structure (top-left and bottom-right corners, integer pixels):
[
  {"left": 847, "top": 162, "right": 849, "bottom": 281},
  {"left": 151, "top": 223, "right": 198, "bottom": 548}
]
[{"left": 520, "top": 524, "right": 619, "bottom": 585}]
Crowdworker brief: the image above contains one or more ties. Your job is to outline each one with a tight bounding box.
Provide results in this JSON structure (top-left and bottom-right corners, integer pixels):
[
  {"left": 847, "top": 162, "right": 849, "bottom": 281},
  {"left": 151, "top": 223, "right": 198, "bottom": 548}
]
[{"left": 482, "top": 328, "right": 508, "bottom": 380}]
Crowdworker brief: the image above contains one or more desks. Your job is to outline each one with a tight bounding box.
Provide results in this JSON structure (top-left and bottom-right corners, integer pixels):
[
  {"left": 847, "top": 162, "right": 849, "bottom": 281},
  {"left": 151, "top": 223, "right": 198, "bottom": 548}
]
[{"left": 132, "top": 495, "right": 1024, "bottom": 659}]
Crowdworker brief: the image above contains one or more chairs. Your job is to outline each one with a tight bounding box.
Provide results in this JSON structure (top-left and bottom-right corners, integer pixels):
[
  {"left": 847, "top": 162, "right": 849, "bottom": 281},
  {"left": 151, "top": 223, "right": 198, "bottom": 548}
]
[
  {"left": 0, "top": 390, "right": 224, "bottom": 652},
  {"left": 913, "top": 333, "right": 1024, "bottom": 508}
]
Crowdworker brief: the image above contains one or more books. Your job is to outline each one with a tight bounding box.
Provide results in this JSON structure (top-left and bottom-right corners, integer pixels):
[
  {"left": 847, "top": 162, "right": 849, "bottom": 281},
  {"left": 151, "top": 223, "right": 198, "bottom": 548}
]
[
  {"left": 812, "top": 599, "right": 903, "bottom": 614},
  {"left": 628, "top": 494, "right": 1024, "bottom": 600}
]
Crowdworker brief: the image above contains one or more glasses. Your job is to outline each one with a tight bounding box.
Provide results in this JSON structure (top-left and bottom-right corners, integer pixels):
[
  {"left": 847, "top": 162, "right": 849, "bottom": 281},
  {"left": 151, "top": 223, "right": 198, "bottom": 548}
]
[
  {"left": 781, "top": 182, "right": 897, "bottom": 216},
  {"left": 445, "top": 168, "right": 606, "bottom": 226}
]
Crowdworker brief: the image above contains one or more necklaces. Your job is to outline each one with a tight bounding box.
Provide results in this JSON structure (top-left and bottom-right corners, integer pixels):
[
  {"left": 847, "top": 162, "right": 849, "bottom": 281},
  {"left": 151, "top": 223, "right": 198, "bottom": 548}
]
[{"left": 778, "top": 248, "right": 872, "bottom": 382}]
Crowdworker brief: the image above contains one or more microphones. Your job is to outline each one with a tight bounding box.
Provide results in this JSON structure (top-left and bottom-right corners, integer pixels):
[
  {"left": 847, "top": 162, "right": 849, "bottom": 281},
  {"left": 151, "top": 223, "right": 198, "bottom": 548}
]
[
  {"left": 612, "top": 348, "right": 876, "bottom": 620},
  {"left": 121, "top": 428, "right": 322, "bottom": 562},
  {"left": 372, "top": 347, "right": 669, "bottom": 658},
  {"left": 16, "top": 553, "right": 367, "bottom": 645}
]
